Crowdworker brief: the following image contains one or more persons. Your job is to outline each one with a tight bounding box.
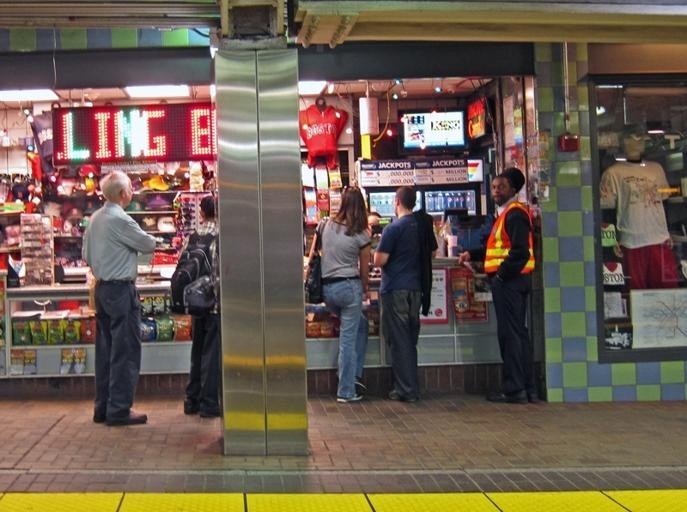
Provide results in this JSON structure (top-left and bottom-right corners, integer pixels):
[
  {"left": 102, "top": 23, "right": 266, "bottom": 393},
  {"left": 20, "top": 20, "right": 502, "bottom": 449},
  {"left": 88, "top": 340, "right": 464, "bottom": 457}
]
[
  {"left": 459, "top": 175, "right": 539, "bottom": 404},
  {"left": 374, "top": 184, "right": 438, "bottom": 402},
  {"left": 365, "top": 212, "right": 382, "bottom": 237},
  {"left": 308, "top": 185, "right": 372, "bottom": 404},
  {"left": 599, "top": 124, "right": 679, "bottom": 291},
  {"left": 82, "top": 171, "right": 157, "bottom": 426},
  {"left": 184, "top": 196, "right": 220, "bottom": 418}
]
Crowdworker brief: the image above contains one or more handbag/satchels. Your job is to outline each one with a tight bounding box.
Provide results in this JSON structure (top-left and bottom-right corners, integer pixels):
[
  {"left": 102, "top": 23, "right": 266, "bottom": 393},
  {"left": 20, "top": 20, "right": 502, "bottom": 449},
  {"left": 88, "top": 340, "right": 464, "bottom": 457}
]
[{"left": 304, "top": 255, "right": 322, "bottom": 303}]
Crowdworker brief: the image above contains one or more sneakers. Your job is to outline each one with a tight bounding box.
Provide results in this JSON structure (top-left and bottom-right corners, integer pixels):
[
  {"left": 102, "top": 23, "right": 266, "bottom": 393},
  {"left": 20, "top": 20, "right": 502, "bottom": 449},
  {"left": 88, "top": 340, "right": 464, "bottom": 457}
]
[
  {"left": 94, "top": 409, "right": 147, "bottom": 426},
  {"left": 184, "top": 404, "right": 221, "bottom": 418},
  {"left": 335, "top": 371, "right": 368, "bottom": 403},
  {"left": 385, "top": 390, "right": 421, "bottom": 403},
  {"left": 487, "top": 389, "right": 540, "bottom": 404},
  {"left": 604, "top": 333, "right": 632, "bottom": 350}
]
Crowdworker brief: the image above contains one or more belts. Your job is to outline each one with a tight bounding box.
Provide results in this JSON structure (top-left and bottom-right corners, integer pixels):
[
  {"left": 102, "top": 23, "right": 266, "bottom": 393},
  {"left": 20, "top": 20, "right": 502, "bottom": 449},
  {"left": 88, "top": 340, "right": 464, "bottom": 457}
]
[
  {"left": 97, "top": 280, "right": 136, "bottom": 285},
  {"left": 324, "top": 274, "right": 359, "bottom": 284}
]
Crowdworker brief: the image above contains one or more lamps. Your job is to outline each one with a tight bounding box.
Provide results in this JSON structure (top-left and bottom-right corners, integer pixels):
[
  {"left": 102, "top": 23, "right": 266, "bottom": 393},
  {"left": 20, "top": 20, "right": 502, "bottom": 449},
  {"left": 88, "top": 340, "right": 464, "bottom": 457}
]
[{"left": 359, "top": 80, "right": 380, "bottom": 136}]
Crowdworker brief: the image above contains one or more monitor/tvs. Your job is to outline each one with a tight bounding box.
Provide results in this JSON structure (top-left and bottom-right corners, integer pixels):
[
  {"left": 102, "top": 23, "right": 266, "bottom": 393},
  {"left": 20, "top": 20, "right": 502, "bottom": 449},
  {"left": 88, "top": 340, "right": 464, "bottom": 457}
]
[{"left": 397, "top": 108, "right": 469, "bottom": 155}]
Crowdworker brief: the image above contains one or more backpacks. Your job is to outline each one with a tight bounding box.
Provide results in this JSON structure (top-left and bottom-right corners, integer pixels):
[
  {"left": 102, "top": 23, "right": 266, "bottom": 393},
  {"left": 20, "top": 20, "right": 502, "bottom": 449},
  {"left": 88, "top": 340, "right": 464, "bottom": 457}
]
[{"left": 171, "top": 230, "right": 220, "bottom": 318}]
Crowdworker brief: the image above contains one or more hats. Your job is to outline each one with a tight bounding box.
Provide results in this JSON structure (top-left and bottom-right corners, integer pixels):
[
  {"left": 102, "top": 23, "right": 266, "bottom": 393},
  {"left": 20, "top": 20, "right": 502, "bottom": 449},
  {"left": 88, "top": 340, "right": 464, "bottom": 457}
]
[
  {"left": 125, "top": 201, "right": 177, "bottom": 233},
  {"left": 11, "top": 184, "right": 27, "bottom": 202},
  {"left": 132, "top": 175, "right": 172, "bottom": 192},
  {"left": 43, "top": 198, "right": 103, "bottom": 238},
  {"left": 78, "top": 163, "right": 104, "bottom": 177},
  {"left": 622, "top": 123, "right": 653, "bottom": 142}
]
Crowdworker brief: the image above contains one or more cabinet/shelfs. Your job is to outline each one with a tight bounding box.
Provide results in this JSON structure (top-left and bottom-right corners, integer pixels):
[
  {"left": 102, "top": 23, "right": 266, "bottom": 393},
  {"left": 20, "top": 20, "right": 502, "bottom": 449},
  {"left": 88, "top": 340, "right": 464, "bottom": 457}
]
[
  {"left": 0, "top": 187, "right": 217, "bottom": 379},
  {"left": 302, "top": 270, "right": 383, "bottom": 370},
  {"left": 300, "top": 144, "right": 355, "bottom": 257}
]
